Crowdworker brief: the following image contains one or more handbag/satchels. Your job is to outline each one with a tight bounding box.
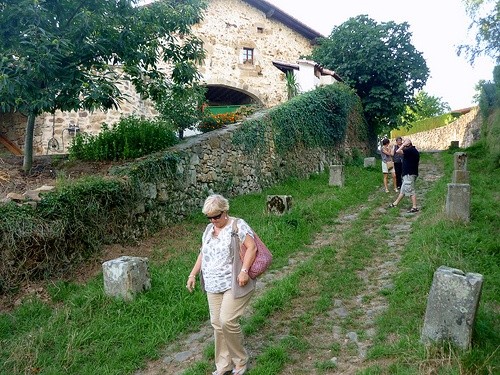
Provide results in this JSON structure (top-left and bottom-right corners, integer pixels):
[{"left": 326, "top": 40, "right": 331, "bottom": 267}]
[
  {"left": 387, "top": 161, "right": 394, "bottom": 170},
  {"left": 240, "top": 232, "right": 272, "bottom": 280}
]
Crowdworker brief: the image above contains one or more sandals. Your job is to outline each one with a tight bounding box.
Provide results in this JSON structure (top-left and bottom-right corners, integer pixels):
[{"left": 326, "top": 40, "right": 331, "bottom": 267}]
[
  {"left": 387, "top": 203, "right": 398, "bottom": 208},
  {"left": 407, "top": 207, "right": 418, "bottom": 213}
]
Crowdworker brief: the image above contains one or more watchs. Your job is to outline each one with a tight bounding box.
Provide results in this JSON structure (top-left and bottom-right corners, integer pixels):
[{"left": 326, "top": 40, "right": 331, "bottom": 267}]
[{"left": 241, "top": 269, "right": 249, "bottom": 273}]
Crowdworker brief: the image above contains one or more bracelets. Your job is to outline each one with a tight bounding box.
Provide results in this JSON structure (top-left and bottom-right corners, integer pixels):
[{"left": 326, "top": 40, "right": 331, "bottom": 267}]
[
  {"left": 188, "top": 276, "right": 196, "bottom": 278},
  {"left": 402, "top": 144, "right": 405, "bottom": 147}
]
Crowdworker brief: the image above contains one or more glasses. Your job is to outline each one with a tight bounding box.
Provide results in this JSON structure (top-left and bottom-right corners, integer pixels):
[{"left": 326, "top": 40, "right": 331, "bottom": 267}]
[{"left": 206, "top": 211, "right": 225, "bottom": 220}]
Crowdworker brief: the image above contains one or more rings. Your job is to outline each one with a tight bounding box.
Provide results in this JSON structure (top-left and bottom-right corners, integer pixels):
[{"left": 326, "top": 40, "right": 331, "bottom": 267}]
[{"left": 186, "top": 285, "right": 188, "bottom": 288}]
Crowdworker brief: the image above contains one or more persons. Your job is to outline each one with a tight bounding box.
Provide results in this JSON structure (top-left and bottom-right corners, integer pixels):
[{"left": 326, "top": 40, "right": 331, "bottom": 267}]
[
  {"left": 393, "top": 137, "right": 404, "bottom": 190},
  {"left": 186, "top": 194, "right": 257, "bottom": 375},
  {"left": 387, "top": 139, "right": 419, "bottom": 213},
  {"left": 381, "top": 139, "right": 399, "bottom": 193}
]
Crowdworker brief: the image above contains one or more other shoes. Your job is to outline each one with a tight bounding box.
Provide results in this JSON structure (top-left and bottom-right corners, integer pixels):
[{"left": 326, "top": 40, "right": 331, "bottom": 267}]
[
  {"left": 212, "top": 363, "right": 234, "bottom": 375},
  {"left": 385, "top": 189, "right": 389, "bottom": 193},
  {"left": 232, "top": 362, "right": 249, "bottom": 375},
  {"left": 393, "top": 188, "right": 399, "bottom": 193}
]
